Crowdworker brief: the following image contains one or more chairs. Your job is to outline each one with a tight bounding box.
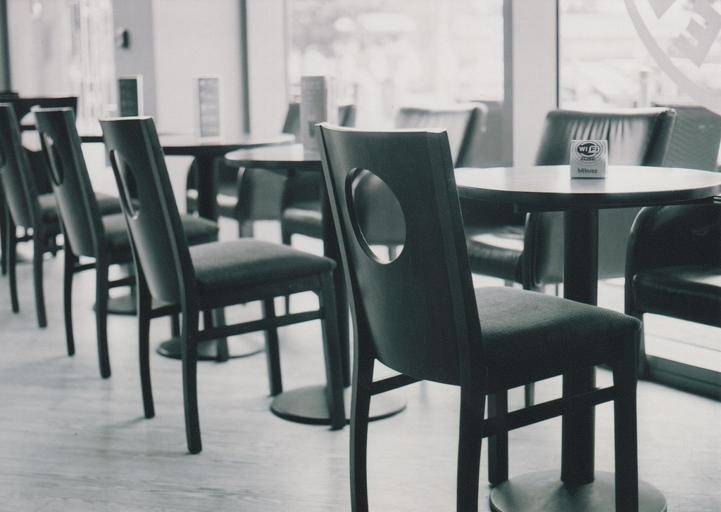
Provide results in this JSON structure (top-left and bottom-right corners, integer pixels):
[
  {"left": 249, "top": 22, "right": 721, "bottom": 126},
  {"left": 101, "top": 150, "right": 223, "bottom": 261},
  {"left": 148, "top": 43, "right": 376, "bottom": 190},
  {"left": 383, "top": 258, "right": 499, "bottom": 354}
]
[{"left": 2, "top": 88, "right": 721, "bottom": 511}]
